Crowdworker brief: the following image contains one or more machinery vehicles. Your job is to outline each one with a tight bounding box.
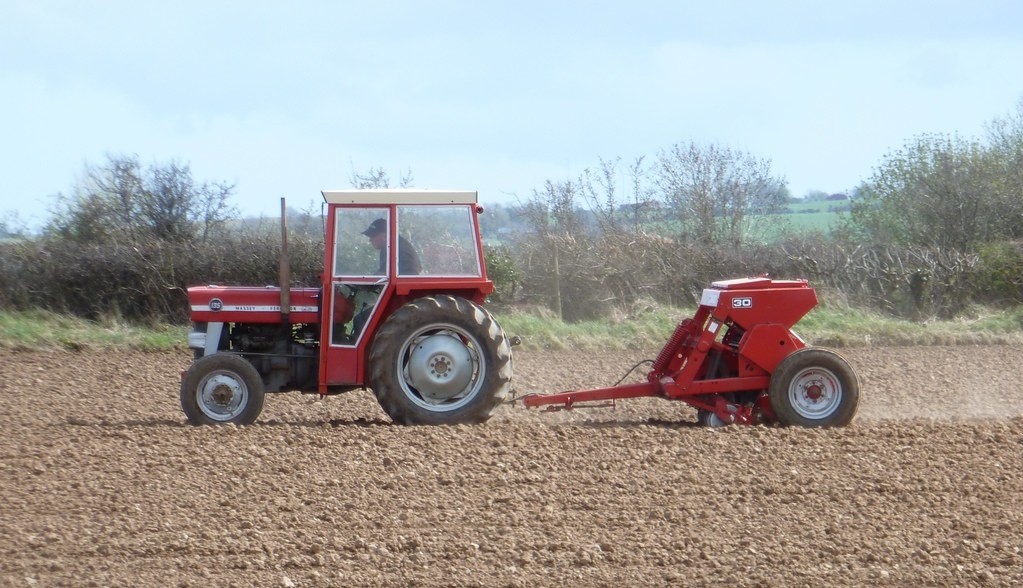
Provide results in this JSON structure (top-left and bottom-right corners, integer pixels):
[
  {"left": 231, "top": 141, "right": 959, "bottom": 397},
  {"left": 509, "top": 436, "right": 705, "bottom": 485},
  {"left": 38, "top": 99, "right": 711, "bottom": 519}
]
[{"left": 178, "top": 187, "right": 861, "bottom": 432}]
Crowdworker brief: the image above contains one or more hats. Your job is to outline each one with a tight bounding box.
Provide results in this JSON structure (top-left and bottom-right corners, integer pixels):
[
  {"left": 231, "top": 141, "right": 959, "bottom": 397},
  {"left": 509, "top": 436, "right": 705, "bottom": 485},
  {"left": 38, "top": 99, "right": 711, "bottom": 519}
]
[{"left": 361, "top": 218, "right": 387, "bottom": 237}]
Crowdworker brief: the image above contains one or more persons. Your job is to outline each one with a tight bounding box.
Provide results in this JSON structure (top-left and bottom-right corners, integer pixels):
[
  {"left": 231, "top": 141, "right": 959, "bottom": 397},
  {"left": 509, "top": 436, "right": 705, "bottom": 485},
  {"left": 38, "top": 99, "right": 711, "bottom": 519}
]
[{"left": 347, "top": 219, "right": 422, "bottom": 343}]
